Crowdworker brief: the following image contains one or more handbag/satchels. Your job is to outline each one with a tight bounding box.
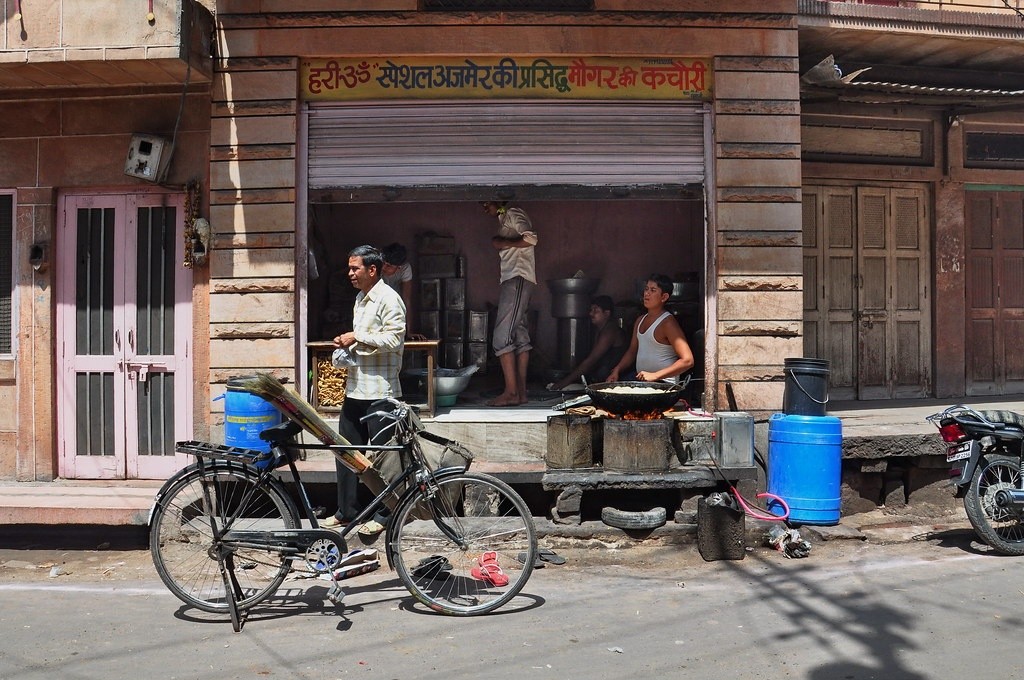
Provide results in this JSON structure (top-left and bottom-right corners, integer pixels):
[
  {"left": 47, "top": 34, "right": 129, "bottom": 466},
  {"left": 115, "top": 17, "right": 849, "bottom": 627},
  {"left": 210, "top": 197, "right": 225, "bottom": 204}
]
[{"left": 354, "top": 404, "right": 474, "bottom": 520}]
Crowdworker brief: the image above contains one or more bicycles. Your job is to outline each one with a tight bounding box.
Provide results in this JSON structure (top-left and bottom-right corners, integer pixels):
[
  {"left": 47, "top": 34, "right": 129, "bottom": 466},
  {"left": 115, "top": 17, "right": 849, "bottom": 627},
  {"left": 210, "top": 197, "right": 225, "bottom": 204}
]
[{"left": 149, "top": 394, "right": 538, "bottom": 633}]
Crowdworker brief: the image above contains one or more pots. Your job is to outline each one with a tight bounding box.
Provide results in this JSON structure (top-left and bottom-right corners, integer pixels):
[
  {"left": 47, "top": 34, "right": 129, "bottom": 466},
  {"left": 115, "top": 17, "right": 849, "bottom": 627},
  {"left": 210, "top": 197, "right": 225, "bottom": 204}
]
[{"left": 581, "top": 373, "right": 691, "bottom": 415}]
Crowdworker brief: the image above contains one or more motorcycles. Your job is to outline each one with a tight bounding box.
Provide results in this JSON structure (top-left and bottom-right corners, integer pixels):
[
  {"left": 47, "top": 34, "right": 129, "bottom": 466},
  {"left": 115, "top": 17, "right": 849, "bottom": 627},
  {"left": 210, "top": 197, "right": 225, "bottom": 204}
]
[{"left": 926, "top": 405, "right": 1024, "bottom": 554}]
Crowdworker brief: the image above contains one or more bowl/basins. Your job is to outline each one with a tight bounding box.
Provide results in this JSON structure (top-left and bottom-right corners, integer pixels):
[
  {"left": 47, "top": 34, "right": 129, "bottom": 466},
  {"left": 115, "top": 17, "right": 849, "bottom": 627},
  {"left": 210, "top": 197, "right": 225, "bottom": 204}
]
[
  {"left": 403, "top": 366, "right": 472, "bottom": 395},
  {"left": 671, "top": 281, "right": 699, "bottom": 301},
  {"left": 546, "top": 277, "right": 605, "bottom": 294},
  {"left": 419, "top": 394, "right": 458, "bottom": 406}
]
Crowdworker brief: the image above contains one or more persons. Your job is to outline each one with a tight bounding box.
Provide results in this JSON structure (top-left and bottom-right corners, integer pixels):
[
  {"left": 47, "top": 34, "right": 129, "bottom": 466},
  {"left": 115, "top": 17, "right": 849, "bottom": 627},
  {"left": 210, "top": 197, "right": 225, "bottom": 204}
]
[
  {"left": 550, "top": 296, "right": 628, "bottom": 391},
  {"left": 381, "top": 242, "right": 427, "bottom": 341},
  {"left": 320, "top": 245, "right": 406, "bottom": 534},
  {"left": 606, "top": 274, "right": 695, "bottom": 386},
  {"left": 481, "top": 202, "right": 538, "bottom": 407}
]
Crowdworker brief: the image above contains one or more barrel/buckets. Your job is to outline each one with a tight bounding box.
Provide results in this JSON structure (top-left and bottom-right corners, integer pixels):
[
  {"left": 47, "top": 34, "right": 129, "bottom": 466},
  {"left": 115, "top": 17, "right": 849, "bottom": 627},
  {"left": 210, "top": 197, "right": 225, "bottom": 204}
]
[
  {"left": 213, "top": 376, "right": 284, "bottom": 469},
  {"left": 559, "top": 318, "right": 592, "bottom": 360},
  {"left": 767, "top": 357, "right": 842, "bottom": 526},
  {"left": 551, "top": 291, "right": 593, "bottom": 320}
]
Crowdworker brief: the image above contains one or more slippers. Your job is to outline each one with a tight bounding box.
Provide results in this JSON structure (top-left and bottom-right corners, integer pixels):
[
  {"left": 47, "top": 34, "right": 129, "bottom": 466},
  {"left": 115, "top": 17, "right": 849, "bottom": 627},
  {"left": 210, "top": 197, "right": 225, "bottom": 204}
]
[
  {"left": 478, "top": 549, "right": 501, "bottom": 567},
  {"left": 472, "top": 564, "right": 509, "bottom": 586},
  {"left": 318, "top": 514, "right": 349, "bottom": 529},
  {"left": 518, "top": 553, "right": 545, "bottom": 567},
  {"left": 410, "top": 566, "right": 451, "bottom": 581},
  {"left": 536, "top": 546, "right": 566, "bottom": 565},
  {"left": 357, "top": 518, "right": 387, "bottom": 535},
  {"left": 419, "top": 554, "right": 454, "bottom": 570}
]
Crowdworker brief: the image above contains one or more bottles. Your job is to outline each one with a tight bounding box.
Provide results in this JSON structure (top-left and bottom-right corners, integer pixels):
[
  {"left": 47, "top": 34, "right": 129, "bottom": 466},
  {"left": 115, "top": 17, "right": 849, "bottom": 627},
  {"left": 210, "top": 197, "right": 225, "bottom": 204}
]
[{"left": 455, "top": 250, "right": 467, "bottom": 278}]
